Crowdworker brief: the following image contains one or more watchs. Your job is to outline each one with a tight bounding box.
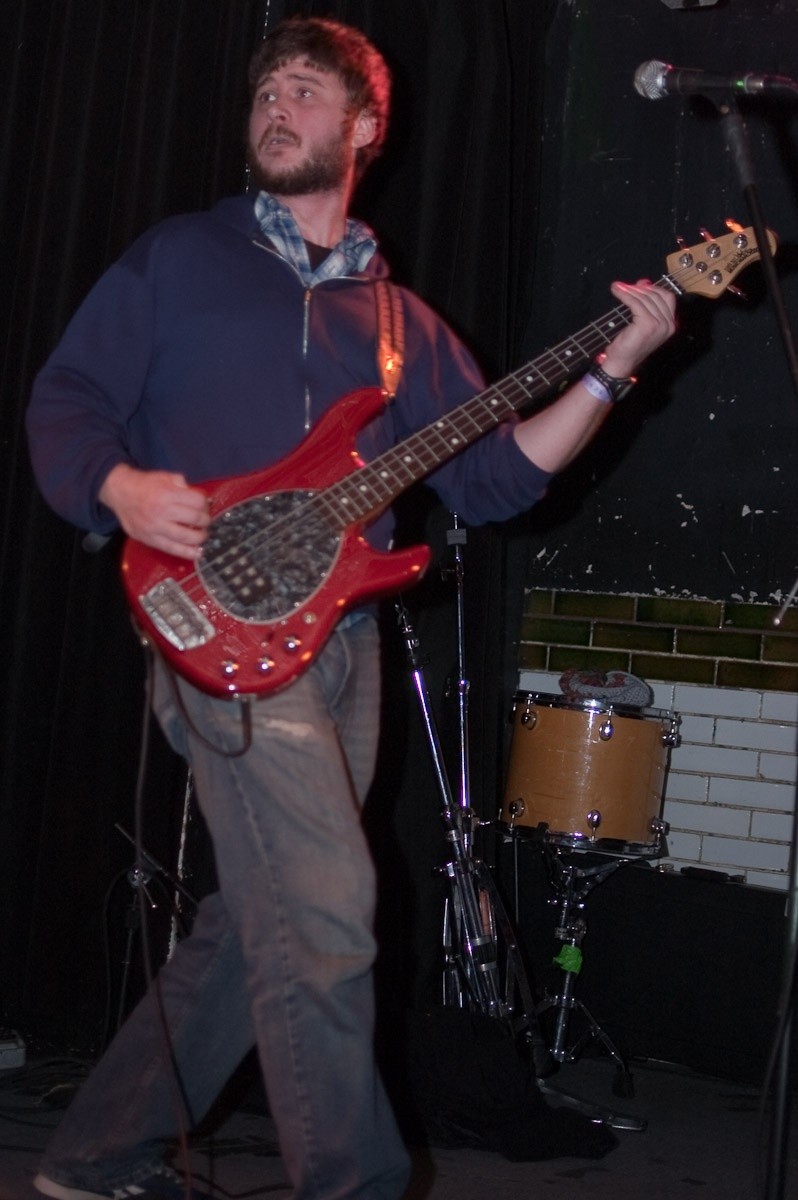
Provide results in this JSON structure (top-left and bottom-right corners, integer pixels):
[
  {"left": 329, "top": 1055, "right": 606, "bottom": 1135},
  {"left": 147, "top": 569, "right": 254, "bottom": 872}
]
[{"left": 590, "top": 361, "right": 637, "bottom": 401}]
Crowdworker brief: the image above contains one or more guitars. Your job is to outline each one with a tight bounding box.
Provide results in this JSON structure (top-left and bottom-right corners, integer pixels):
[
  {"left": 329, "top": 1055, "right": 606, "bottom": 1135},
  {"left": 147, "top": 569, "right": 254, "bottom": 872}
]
[{"left": 121, "top": 220, "right": 779, "bottom": 700}]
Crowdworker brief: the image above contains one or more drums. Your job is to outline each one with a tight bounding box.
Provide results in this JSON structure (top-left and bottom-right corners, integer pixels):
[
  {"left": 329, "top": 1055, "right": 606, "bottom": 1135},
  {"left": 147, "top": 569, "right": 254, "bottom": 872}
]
[{"left": 498, "top": 689, "right": 682, "bottom": 859}]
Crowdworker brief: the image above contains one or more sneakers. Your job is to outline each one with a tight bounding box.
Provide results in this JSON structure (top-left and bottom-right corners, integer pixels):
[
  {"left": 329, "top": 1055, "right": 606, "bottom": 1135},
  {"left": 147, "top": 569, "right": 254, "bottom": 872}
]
[{"left": 33, "top": 1152, "right": 203, "bottom": 1200}]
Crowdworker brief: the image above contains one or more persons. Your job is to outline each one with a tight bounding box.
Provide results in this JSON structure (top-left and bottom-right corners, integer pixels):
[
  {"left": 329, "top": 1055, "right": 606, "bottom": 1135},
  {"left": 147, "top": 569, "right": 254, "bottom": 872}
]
[{"left": 23, "top": 14, "right": 680, "bottom": 1197}]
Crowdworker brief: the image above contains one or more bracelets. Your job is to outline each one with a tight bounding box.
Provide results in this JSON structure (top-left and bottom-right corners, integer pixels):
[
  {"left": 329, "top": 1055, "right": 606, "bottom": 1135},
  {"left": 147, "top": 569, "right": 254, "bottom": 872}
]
[{"left": 584, "top": 374, "right": 610, "bottom": 403}]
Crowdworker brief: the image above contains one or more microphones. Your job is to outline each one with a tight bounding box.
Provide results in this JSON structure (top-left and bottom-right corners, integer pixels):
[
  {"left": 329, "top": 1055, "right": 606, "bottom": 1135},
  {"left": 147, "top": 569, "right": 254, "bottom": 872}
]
[{"left": 634, "top": 60, "right": 791, "bottom": 102}]
